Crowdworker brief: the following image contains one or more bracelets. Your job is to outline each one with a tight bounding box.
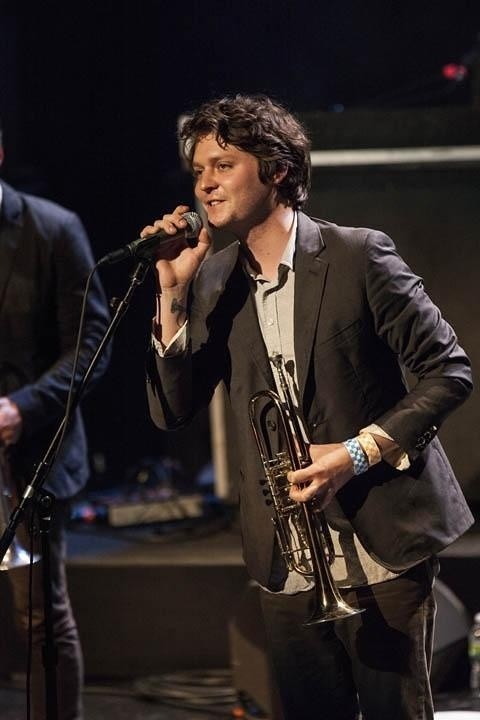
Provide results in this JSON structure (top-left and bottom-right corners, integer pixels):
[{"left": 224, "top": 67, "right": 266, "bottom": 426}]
[
  {"left": 355, "top": 432, "right": 382, "bottom": 468},
  {"left": 343, "top": 438, "right": 369, "bottom": 476}
]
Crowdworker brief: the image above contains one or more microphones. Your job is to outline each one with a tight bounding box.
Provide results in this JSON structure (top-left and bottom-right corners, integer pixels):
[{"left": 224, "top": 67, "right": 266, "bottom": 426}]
[{"left": 106, "top": 212, "right": 203, "bottom": 266}]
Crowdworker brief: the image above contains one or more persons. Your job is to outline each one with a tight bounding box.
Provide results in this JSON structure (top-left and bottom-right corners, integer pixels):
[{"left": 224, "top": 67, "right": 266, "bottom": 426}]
[
  {"left": 0, "top": 131, "right": 115, "bottom": 720},
  {"left": 141, "top": 94, "right": 480, "bottom": 720}
]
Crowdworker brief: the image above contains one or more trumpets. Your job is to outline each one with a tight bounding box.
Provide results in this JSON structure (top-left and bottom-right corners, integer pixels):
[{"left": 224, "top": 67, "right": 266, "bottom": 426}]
[
  {"left": 1, "top": 441, "right": 42, "bottom": 570},
  {"left": 248, "top": 354, "right": 365, "bottom": 627}
]
